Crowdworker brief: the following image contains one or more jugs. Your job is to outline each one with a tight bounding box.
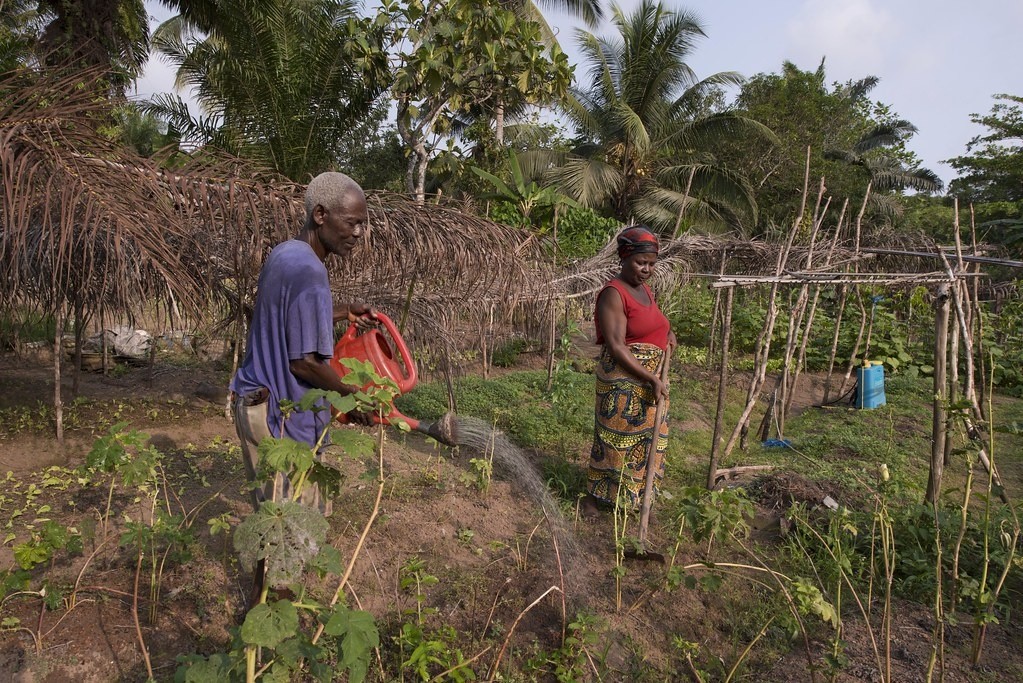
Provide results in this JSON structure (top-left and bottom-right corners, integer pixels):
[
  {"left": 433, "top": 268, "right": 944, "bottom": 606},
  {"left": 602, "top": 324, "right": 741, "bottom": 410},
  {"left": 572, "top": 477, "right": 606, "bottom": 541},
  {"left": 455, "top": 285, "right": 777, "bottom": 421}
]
[{"left": 329, "top": 312, "right": 457, "bottom": 446}]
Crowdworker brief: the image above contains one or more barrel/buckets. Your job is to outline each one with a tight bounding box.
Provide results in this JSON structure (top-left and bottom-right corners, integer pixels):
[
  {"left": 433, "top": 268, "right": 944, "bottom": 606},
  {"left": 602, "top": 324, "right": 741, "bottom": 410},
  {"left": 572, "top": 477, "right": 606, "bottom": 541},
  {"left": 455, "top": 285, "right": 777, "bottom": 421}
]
[{"left": 857, "top": 360, "right": 885, "bottom": 409}]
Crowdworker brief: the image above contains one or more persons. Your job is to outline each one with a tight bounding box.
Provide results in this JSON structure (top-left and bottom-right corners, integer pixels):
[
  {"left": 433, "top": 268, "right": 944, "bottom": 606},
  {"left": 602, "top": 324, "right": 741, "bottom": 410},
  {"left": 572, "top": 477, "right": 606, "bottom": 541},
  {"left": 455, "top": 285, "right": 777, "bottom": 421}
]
[
  {"left": 231, "top": 172, "right": 386, "bottom": 616},
  {"left": 588, "top": 225, "right": 675, "bottom": 524}
]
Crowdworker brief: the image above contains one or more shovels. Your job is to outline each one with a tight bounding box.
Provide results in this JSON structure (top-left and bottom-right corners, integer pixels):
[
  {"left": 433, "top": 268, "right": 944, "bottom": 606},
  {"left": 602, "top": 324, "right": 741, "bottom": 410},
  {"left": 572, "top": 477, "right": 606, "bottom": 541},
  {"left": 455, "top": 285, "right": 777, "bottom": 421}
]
[{"left": 622, "top": 343, "right": 671, "bottom": 565}]
